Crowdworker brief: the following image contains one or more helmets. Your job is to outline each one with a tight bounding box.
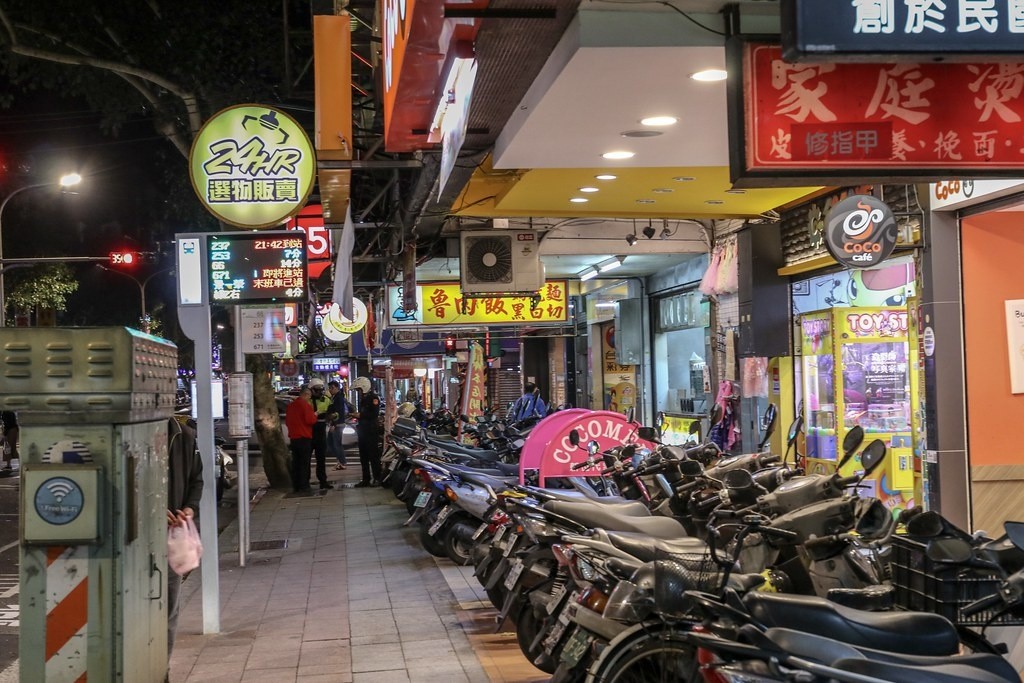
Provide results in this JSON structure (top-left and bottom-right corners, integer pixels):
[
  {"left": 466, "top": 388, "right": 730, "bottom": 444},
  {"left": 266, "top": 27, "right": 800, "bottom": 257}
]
[
  {"left": 308, "top": 378, "right": 324, "bottom": 388},
  {"left": 601, "top": 560, "right": 695, "bottom": 628},
  {"left": 349, "top": 377, "right": 371, "bottom": 394},
  {"left": 398, "top": 402, "right": 417, "bottom": 418}
]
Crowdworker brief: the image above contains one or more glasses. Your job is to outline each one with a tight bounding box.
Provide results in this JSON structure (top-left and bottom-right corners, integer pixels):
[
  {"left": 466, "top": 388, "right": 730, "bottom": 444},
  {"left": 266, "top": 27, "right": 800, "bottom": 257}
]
[{"left": 356, "top": 388, "right": 361, "bottom": 391}]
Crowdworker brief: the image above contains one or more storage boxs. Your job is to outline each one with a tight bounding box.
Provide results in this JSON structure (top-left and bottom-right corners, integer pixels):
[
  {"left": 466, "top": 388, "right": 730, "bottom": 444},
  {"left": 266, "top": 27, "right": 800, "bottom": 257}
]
[{"left": 890, "top": 533, "right": 1024, "bottom": 627}]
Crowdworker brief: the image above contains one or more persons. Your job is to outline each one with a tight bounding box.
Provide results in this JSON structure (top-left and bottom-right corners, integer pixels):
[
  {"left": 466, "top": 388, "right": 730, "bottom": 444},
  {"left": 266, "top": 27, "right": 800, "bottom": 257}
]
[
  {"left": 167, "top": 416, "right": 204, "bottom": 659},
  {"left": 306, "top": 378, "right": 335, "bottom": 489},
  {"left": 513, "top": 382, "right": 545, "bottom": 421},
  {"left": 327, "top": 380, "right": 348, "bottom": 470},
  {"left": 2, "top": 410, "right": 20, "bottom": 469},
  {"left": 348, "top": 377, "right": 381, "bottom": 488},
  {"left": 286, "top": 387, "right": 317, "bottom": 494}
]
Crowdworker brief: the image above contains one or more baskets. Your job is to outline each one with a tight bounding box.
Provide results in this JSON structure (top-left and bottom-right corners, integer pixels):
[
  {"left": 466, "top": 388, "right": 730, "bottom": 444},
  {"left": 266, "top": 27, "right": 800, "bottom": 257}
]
[
  {"left": 655, "top": 552, "right": 722, "bottom": 621},
  {"left": 891, "top": 534, "right": 1024, "bottom": 626}
]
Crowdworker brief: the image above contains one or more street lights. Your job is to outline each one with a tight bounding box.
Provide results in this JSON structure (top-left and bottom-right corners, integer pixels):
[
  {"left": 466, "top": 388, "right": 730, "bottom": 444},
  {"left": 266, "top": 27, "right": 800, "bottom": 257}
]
[
  {"left": 0, "top": 173, "right": 82, "bottom": 328},
  {"left": 96, "top": 263, "right": 174, "bottom": 333}
]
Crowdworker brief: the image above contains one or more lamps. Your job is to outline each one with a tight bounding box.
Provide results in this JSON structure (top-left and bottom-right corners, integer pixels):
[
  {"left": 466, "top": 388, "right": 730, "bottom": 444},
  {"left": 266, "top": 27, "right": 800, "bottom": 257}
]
[
  {"left": 577, "top": 255, "right": 627, "bottom": 282},
  {"left": 643, "top": 218, "right": 655, "bottom": 238},
  {"left": 626, "top": 220, "right": 638, "bottom": 246},
  {"left": 659, "top": 219, "right": 671, "bottom": 240}
]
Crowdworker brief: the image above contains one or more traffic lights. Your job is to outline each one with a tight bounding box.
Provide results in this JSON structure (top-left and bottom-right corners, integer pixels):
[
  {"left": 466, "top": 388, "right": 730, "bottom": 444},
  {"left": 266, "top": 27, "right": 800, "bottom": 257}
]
[{"left": 110, "top": 252, "right": 138, "bottom": 266}]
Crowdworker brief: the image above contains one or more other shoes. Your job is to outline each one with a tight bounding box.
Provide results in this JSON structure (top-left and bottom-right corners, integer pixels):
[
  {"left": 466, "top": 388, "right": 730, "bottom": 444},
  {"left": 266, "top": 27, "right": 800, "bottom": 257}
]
[
  {"left": 355, "top": 480, "right": 371, "bottom": 488},
  {"left": 294, "top": 489, "right": 300, "bottom": 494},
  {"left": 370, "top": 481, "right": 377, "bottom": 487},
  {"left": 333, "top": 465, "right": 346, "bottom": 470},
  {"left": 303, "top": 487, "right": 312, "bottom": 492},
  {"left": 320, "top": 483, "right": 333, "bottom": 489},
  {"left": 332, "top": 463, "right": 340, "bottom": 468}
]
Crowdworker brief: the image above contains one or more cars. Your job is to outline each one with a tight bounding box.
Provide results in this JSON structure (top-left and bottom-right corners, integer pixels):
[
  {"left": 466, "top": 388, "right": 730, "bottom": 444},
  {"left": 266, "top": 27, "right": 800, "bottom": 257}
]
[
  {"left": 173, "top": 406, "right": 191, "bottom": 426},
  {"left": 214, "top": 395, "right": 359, "bottom": 456}
]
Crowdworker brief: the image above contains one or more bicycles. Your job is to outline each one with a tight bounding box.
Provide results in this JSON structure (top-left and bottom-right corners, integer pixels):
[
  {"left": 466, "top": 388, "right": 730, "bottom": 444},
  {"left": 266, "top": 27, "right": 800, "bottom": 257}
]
[{"left": 604, "top": 499, "right": 1003, "bottom": 683}]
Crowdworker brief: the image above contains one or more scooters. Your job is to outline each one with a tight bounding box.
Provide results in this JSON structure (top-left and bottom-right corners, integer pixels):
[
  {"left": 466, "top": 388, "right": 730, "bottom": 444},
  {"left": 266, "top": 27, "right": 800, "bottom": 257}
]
[{"left": 380, "top": 393, "right": 1024, "bottom": 683}]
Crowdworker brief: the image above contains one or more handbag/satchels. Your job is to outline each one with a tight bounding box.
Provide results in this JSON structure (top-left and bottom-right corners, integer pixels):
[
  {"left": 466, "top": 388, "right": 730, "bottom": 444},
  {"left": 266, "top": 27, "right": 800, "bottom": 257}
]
[{"left": 167, "top": 516, "right": 203, "bottom": 577}]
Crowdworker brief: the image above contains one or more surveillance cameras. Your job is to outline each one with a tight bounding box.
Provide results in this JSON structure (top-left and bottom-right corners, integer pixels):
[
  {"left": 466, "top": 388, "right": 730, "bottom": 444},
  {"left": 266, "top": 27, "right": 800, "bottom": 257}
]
[{"left": 660, "top": 229, "right": 671, "bottom": 240}]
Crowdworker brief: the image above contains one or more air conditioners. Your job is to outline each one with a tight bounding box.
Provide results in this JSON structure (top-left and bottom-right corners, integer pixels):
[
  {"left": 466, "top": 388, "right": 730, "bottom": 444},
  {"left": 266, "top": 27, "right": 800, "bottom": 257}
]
[{"left": 460, "top": 230, "right": 539, "bottom": 293}]
